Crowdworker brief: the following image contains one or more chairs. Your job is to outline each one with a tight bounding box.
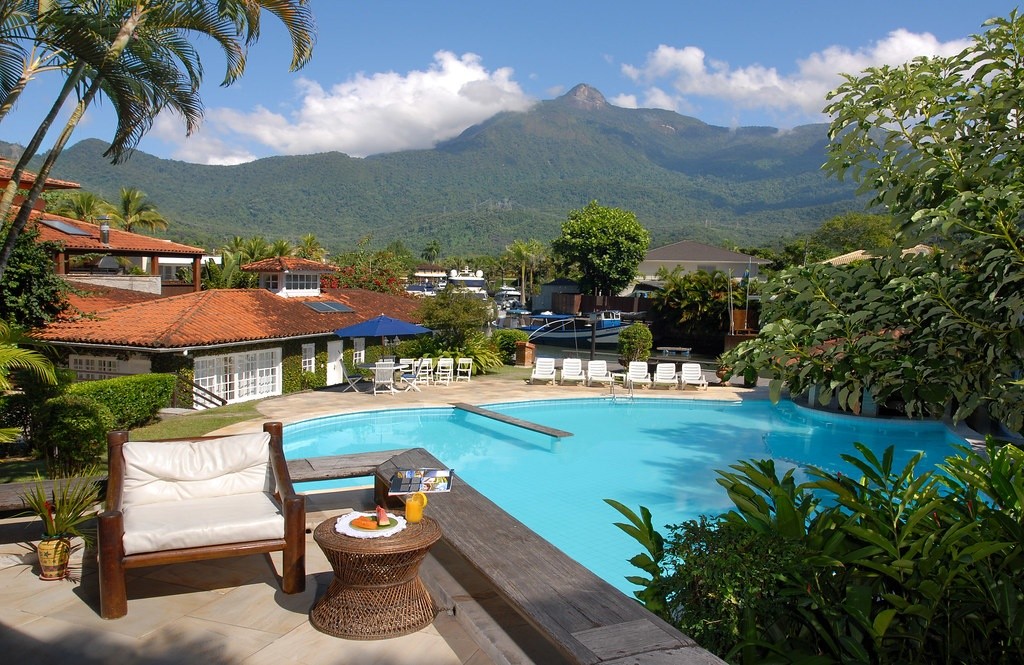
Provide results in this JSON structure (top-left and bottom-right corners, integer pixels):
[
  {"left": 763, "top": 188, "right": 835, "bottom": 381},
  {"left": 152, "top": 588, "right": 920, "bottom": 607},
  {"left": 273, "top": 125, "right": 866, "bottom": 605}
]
[
  {"left": 629, "top": 361, "right": 652, "bottom": 391},
  {"left": 681, "top": 363, "right": 708, "bottom": 390},
  {"left": 530, "top": 357, "right": 556, "bottom": 386},
  {"left": 337, "top": 350, "right": 473, "bottom": 396},
  {"left": 587, "top": 359, "right": 612, "bottom": 388},
  {"left": 654, "top": 363, "right": 678, "bottom": 390},
  {"left": 94, "top": 420, "right": 308, "bottom": 620},
  {"left": 560, "top": 358, "right": 585, "bottom": 385}
]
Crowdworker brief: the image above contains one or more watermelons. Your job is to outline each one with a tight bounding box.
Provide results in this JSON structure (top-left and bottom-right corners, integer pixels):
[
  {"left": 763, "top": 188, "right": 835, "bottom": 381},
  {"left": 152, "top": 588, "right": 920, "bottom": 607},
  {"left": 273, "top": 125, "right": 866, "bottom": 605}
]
[{"left": 376, "top": 505, "right": 391, "bottom": 525}]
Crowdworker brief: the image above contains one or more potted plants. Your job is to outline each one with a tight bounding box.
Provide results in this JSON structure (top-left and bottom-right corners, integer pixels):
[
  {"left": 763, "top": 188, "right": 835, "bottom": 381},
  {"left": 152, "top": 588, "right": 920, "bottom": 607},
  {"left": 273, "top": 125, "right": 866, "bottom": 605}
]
[{"left": 6, "top": 464, "right": 108, "bottom": 580}]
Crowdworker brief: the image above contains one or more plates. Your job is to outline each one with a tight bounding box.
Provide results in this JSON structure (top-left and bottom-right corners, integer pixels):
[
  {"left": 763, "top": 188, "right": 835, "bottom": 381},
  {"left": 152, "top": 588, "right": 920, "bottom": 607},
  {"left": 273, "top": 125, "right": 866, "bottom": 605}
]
[{"left": 349, "top": 516, "right": 398, "bottom": 532}]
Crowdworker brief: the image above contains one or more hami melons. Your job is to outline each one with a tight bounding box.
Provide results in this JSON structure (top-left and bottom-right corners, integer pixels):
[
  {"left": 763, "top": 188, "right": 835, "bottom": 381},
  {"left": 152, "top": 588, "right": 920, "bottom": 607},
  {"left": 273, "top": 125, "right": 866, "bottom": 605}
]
[{"left": 351, "top": 516, "right": 378, "bottom": 529}]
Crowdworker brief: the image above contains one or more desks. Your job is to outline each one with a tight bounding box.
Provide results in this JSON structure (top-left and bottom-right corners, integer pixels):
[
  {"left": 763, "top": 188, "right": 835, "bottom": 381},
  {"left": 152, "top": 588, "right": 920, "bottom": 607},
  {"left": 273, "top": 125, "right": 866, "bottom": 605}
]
[
  {"left": 358, "top": 362, "right": 410, "bottom": 392},
  {"left": 611, "top": 372, "right": 626, "bottom": 387},
  {"left": 310, "top": 509, "right": 443, "bottom": 640}
]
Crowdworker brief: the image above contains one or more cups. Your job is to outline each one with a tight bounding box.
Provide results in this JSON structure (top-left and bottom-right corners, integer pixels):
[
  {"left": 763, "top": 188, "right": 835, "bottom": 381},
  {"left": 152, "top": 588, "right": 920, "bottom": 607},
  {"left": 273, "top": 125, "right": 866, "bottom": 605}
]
[{"left": 405, "top": 492, "right": 423, "bottom": 524}]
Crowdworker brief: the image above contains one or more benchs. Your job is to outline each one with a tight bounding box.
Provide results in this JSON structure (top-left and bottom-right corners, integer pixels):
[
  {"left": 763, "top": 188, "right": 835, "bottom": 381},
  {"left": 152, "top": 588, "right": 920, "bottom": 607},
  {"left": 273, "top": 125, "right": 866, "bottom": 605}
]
[{"left": 657, "top": 347, "right": 692, "bottom": 359}]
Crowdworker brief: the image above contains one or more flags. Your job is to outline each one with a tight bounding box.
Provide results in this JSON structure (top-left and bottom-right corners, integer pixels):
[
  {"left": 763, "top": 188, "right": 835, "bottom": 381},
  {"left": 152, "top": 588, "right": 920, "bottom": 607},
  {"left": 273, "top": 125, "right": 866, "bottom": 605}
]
[{"left": 741, "top": 264, "right": 750, "bottom": 287}]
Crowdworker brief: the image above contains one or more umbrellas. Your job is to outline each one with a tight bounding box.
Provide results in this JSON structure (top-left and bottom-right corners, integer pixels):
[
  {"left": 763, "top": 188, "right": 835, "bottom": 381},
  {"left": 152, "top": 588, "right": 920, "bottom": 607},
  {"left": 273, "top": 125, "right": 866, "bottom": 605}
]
[{"left": 334, "top": 313, "right": 433, "bottom": 362}]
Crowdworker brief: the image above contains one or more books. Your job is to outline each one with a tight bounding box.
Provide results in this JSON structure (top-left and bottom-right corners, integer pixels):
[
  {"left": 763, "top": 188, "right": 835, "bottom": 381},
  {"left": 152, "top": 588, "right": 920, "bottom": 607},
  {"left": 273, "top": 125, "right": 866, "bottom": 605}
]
[{"left": 388, "top": 465, "right": 454, "bottom": 496}]
